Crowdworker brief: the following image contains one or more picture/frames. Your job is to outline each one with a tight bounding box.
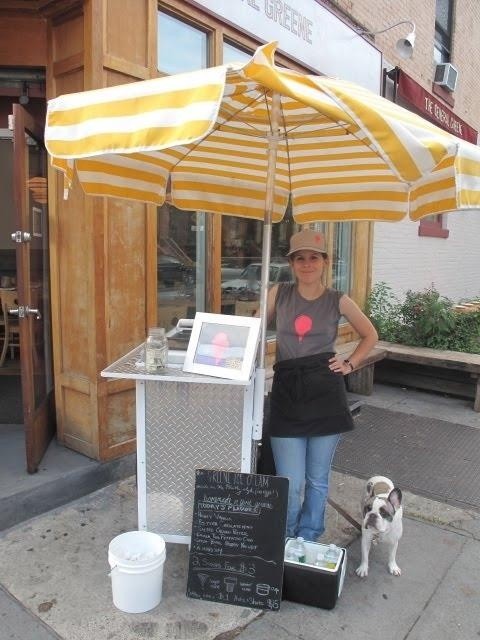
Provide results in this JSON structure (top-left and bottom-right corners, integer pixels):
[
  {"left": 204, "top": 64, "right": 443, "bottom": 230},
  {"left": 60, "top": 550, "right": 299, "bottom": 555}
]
[{"left": 182, "top": 311, "right": 262, "bottom": 383}]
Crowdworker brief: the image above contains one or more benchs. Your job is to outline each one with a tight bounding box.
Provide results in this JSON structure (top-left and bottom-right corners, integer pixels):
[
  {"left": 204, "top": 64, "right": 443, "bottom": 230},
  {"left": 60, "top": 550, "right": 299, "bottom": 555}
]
[
  {"left": 374, "top": 340, "right": 480, "bottom": 412},
  {"left": 266, "top": 338, "right": 387, "bottom": 395}
]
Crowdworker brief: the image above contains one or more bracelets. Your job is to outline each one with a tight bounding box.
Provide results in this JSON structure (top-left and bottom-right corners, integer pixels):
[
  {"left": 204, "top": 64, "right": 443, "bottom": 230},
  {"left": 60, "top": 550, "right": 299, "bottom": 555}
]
[{"left": 345, "top": 359, "right": 354, "bottom": 371}]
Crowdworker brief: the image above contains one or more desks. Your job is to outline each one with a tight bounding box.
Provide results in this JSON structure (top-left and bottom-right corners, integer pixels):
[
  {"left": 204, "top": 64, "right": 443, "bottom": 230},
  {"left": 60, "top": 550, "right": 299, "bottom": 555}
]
[{"left": 100, "top": 328, "right": 259, "bottom": 546}]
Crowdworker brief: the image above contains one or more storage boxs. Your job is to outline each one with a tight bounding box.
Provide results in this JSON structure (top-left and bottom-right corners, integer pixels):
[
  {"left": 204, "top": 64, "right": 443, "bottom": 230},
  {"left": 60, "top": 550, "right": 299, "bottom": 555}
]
[{"left": 282, "top": 537, "right": 347, "bottom": 611}]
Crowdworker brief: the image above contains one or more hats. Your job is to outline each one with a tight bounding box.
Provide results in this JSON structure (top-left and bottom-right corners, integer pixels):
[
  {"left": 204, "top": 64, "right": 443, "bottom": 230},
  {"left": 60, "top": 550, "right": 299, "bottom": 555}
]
[{"left": 285, "top": 228, "right": 328, "bottom": 258}]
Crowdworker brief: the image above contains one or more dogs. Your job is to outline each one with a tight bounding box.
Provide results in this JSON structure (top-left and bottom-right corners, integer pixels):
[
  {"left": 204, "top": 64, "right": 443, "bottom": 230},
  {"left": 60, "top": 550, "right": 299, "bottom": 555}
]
[{"left": 354, "top": 475, "right": 404, "bottom": 578}]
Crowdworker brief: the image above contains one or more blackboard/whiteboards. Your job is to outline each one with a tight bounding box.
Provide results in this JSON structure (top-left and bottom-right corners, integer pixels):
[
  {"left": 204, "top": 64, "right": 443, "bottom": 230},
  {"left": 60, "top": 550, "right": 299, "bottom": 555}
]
[{"left": 186, "top": 469, "right": 289, "bottom": 611}]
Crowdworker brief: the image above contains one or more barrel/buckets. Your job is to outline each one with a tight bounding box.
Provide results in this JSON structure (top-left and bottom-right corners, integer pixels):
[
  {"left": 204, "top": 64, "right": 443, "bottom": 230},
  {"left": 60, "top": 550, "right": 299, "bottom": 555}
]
[
  {"left": 146, "top": 495, "right": 184, "bottom": 537},
  {"left": 106, "top": 531, "right": 166, "bottom": 613}
]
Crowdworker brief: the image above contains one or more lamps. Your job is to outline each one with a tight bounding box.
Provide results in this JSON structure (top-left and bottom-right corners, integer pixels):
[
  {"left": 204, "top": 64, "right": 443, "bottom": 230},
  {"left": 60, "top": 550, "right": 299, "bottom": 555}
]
[{"left": 362, "top": 20, "right": 416, "bottom": 59}]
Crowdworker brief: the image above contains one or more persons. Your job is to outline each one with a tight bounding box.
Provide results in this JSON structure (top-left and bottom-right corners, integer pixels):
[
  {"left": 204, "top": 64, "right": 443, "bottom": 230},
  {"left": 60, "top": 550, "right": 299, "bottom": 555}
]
[{"left": 255, "top": 229, "right": 379, "bottom": 546}]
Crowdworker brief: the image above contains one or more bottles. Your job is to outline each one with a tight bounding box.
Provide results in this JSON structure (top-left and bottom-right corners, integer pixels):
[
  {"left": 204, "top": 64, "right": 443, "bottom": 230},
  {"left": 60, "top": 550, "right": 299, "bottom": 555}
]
[
  {"left": 145, "top": 327, "right": 167, "bottom": 375},
  {"left": 287, "top": 536, "right": 338, "bottom": 570}
]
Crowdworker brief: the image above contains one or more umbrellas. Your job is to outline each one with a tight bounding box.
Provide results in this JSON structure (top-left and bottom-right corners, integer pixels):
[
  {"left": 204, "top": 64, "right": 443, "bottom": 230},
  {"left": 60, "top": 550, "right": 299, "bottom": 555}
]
[{"left": 44, "top": 39, "right": 479, "bottom": 374}]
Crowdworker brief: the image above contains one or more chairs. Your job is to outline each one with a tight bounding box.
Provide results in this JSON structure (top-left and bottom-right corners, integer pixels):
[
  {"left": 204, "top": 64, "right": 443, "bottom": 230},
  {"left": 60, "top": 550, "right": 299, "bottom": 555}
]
[{"left": 0, "top": 275, "right": 42, "bottom": 367}]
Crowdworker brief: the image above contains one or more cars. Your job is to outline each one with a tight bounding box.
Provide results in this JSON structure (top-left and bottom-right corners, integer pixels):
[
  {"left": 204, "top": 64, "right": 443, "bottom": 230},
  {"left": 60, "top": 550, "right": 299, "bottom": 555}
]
[{"left": 158, "top": 255, "right": 336, "bottom": 303}]
